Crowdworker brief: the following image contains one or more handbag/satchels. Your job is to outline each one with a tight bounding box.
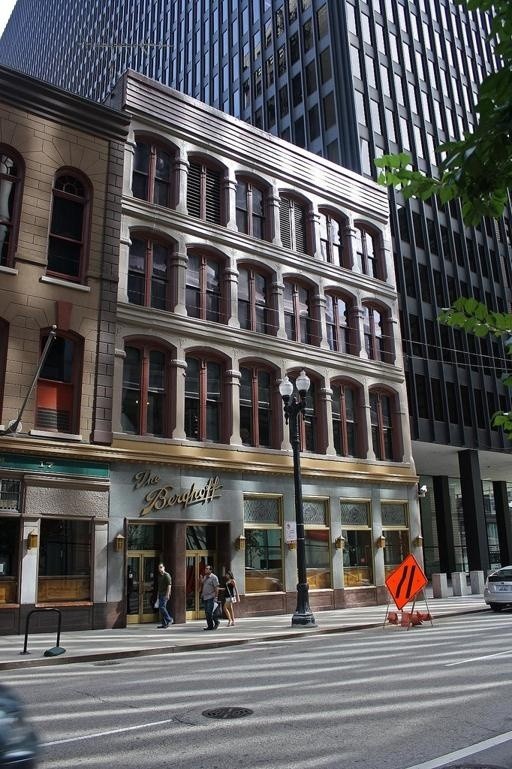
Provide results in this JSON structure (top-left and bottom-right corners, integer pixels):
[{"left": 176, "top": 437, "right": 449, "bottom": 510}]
[
  {"left": 231, "top": 597, "right": 237, "bottom": 603},
  {"left": 212, "top": 602, "right": 220, "bottom": 617}
]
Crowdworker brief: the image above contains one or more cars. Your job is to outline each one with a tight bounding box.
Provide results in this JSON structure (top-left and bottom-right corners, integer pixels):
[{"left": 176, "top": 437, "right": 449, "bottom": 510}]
[{"left": 484, "top": 565, "right": 512, "bottom": 610}]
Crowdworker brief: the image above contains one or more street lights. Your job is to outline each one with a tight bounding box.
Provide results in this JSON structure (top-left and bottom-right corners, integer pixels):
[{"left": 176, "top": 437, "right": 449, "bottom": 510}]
[{"left": 279, "top": 371, "right": 316, "bottom": 628}]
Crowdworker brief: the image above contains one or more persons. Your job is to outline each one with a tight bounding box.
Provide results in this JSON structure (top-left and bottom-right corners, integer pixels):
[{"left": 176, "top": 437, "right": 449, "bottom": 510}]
[
  {"left": 218, "top": 570, "right": 240, "bottom": 628},
  {"left": 198, "top": 565, "right": 221, "bottom": 632},
  {"left": 154, "top": 561, "right": 174, "bottom": 629}
]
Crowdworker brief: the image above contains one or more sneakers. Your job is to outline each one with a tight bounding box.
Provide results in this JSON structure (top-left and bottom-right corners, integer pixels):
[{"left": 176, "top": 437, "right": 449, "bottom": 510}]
[
  {"left": 227, "top": 619, "right": 235, "bottom": 627},
  {"left": 203, "top": 621, "right": 220, "bottom": 630},
  {"left": 157, "top": 619, "right": 173, "bottom": 628}
]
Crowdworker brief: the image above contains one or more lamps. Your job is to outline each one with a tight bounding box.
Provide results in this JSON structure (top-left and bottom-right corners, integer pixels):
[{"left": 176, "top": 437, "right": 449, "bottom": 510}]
[
  {"left": 289, "top": 540, "right": 295, "bottom": 550},
  {"left": 27, "top": 529, "right": 37, "bottom": 550},
  {"left": 336, "top": 536, "right": 345, "bottom": 550},
  {"left": 237, "top": 534, "right": 246, "bottom": 550},
  {"left": 377, "top": 535, "right": 385, "bottom": 548},
  {"left": 415, "top": 534, "right": 423, "bottom": 547},
  {"left": 115, "top": 534, "right": 125, "bottom": 552}
]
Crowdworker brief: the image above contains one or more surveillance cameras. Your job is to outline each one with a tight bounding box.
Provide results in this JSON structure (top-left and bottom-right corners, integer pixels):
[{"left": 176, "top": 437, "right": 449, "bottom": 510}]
[{"left": 420, "top": 485, "right": 427, "bottom": 492}]
